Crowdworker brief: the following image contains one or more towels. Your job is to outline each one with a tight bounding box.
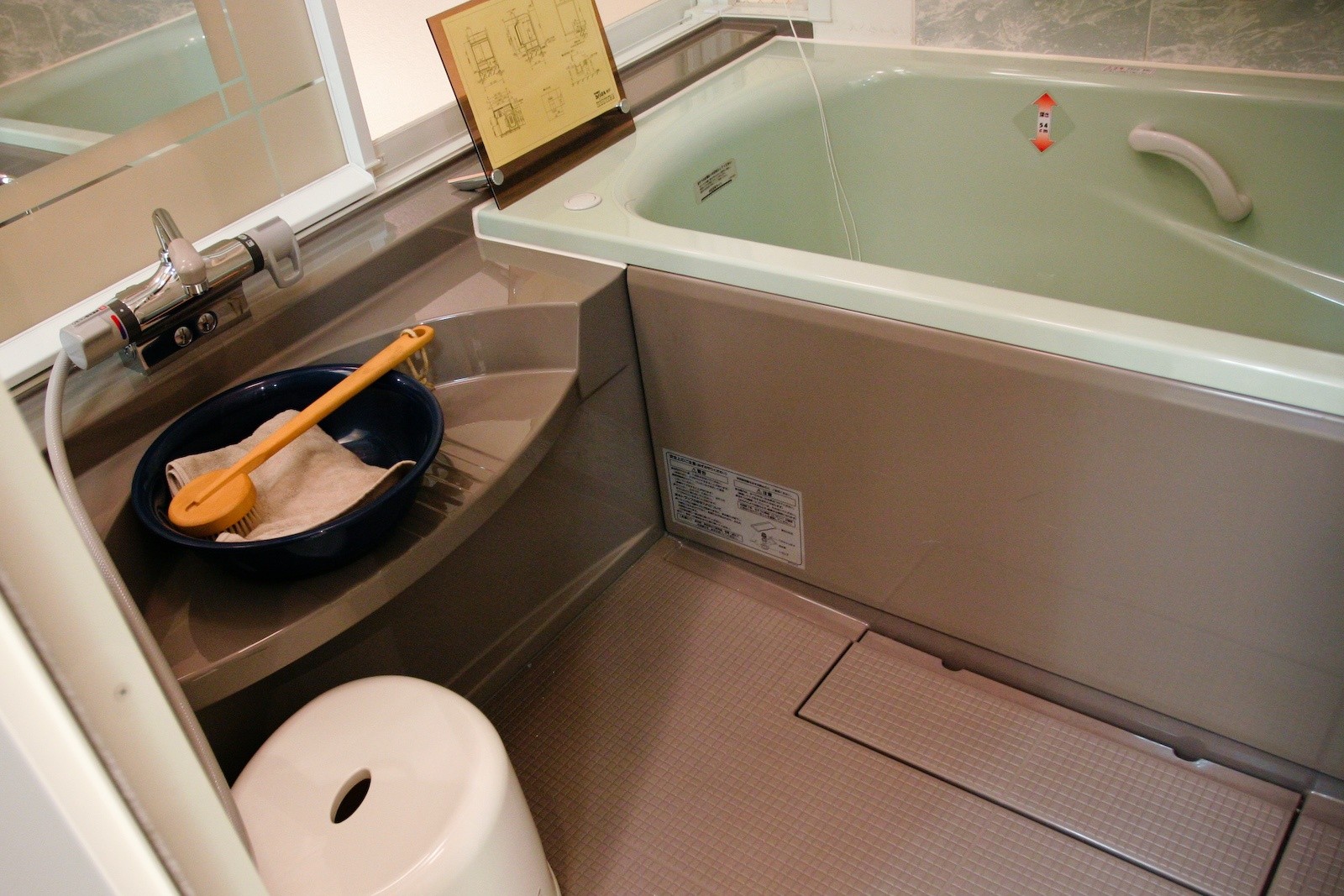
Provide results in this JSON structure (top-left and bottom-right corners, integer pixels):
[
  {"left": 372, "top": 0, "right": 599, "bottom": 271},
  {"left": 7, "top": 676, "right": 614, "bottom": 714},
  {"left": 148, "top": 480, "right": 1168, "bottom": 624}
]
[{"left": 166, "top": 407, "right": 418, "bottom": 543}]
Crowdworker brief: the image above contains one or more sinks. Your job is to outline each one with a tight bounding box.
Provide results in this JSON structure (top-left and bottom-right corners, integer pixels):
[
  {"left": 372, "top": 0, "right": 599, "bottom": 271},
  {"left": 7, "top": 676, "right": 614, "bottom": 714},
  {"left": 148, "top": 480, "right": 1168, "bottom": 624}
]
[{"left": 126, "top": 359, "right": 445, "bottom": 588}]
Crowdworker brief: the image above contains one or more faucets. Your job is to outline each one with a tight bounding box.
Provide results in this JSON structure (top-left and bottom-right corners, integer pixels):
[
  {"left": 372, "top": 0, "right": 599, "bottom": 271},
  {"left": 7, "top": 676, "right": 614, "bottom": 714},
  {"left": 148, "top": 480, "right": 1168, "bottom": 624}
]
[{"left": 149, "top": 206, "right": 212, "bottom": 297}]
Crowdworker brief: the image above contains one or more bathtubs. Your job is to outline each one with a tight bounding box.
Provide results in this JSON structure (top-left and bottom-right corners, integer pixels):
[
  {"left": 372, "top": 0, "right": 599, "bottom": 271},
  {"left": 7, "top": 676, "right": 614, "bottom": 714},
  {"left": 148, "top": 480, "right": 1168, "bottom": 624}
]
[{"left": 470, "top": 29, "right": 1344, "bottom": 808}]
